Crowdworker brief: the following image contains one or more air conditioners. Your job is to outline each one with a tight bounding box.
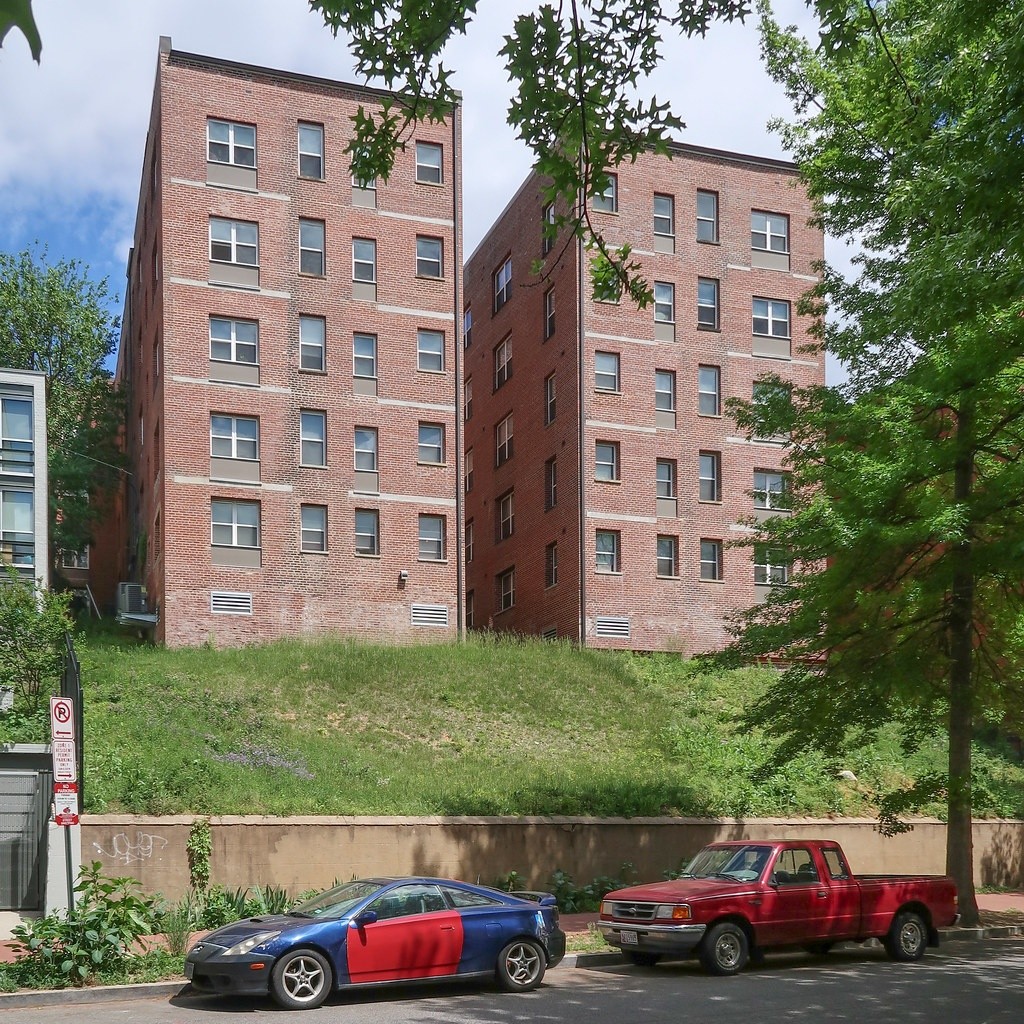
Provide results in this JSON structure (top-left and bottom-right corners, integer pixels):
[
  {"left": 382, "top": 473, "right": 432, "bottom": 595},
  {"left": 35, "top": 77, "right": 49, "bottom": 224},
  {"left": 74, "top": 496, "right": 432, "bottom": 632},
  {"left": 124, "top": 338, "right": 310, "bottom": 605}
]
[{"left": 115, "top": 582, "right": 147, "bottom": 614}]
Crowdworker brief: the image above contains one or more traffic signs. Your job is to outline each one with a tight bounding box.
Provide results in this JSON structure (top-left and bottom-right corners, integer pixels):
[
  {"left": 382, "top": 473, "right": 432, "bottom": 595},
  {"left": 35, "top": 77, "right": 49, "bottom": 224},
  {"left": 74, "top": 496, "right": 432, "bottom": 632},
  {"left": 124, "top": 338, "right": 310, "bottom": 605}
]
[{"left": 51, "top": 739, "right": 77, "bottom": 782}]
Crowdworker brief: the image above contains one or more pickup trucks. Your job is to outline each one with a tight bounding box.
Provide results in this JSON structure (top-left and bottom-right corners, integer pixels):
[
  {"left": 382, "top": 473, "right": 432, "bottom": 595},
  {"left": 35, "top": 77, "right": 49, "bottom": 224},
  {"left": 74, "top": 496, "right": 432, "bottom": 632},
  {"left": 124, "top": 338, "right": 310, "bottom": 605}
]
[{"left": 598, "top": 839, "right": 959, "bottom": 971}]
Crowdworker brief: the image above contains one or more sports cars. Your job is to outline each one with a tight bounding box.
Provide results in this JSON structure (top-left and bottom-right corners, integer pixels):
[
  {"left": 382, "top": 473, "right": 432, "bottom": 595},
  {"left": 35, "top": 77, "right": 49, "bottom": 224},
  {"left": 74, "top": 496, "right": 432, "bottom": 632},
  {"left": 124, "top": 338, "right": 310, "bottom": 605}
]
[{"left": 183, "top": 878, "right": 565, "bottom": 1007}]
